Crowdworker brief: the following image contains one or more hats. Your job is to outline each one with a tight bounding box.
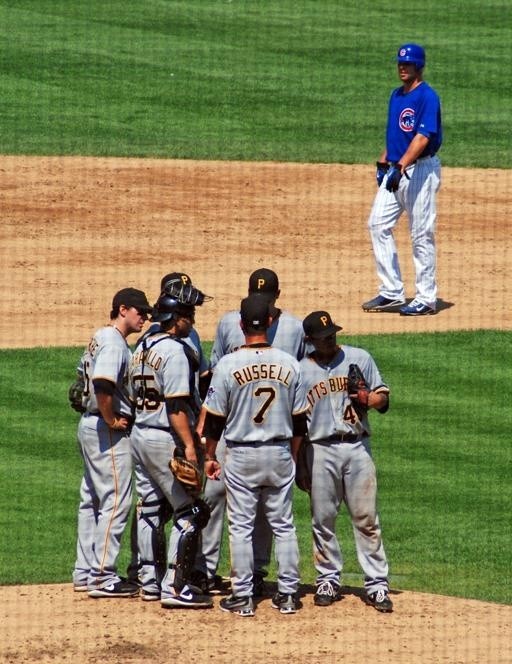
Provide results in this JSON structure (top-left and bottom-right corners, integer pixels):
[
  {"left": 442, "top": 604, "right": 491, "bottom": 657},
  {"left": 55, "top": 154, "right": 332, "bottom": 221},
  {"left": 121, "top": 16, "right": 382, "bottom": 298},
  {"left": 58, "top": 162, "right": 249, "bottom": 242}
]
[
  {"left": 112, "top": 287, "right": 154, "bottom": 312},
  {"left": 162, "top": 272, "right": 190, "bottom": 288},
  {"left": 240, "top": 295, "right": 278, "bottom": 327},
  {"left": 303, "top": 310, "right": 342, "bottom": 338},
  {"left": 248, "top": 267, "right": 278, "bottom": 300}
]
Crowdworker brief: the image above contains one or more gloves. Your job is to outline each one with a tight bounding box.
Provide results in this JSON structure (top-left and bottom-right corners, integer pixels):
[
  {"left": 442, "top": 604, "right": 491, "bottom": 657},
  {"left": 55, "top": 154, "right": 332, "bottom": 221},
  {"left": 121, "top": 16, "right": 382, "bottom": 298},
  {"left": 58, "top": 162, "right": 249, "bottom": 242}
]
[
  {"left": 376, "top": 161, "right": 390, "bottom": 187},
  {"left": 385, "top": 164, "right": 401, "bottom": 193}
]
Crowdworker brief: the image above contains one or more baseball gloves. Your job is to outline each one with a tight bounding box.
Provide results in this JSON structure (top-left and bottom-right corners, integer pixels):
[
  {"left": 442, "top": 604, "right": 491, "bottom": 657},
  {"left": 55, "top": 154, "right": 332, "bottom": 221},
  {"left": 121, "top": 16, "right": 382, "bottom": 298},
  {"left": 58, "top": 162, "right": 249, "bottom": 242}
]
[
  {"left": 347, "top": 364, "right": 367, "bottom": 418},
  {"left": 168, "top": 447, "right": 205, "bottom": 492}
]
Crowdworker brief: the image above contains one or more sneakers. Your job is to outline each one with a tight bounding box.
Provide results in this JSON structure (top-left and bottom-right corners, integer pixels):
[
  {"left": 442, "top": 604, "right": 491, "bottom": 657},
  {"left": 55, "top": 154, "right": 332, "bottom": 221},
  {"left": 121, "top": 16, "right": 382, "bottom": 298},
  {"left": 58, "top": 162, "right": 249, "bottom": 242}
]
[
  {"left": 219, "top": 594, "right": 256, "bottom": 616},
  {"left": 365, "top": 590, "right": 393, "bottom": 613},
  {"left": 362, "top": 296, "right": 406, "bottom": 312},
  {"left": 140, "top": 588, "right": 160, "bottom": 602},
  {"left": 271, "top": 592, "right": 299, "bottom": 613},
  {"left": 400, "top": 299, "right": 436, "bottom": 317},
  {"left": 73, "top": 585, "right": 88, "bottom": 592},
  {"left": 87, "top": 577, "right": 139, "bottom": 596},
  {"left": 161, "top": 592, "right": 213, "bottom": 608},
  {"left": 314, "top": 582, "right": 341, "bottom": 606},
  {"left": 209, "top": 577, "right": 233, "bottom": 594}
]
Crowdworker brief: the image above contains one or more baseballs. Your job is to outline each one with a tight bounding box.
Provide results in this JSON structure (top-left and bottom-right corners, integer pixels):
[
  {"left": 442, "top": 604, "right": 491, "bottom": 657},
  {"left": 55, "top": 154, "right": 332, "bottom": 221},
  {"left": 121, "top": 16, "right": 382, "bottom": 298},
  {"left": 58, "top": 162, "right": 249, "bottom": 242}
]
[{"left": 200, "top": 437, "right": 207, "bottom": 444}]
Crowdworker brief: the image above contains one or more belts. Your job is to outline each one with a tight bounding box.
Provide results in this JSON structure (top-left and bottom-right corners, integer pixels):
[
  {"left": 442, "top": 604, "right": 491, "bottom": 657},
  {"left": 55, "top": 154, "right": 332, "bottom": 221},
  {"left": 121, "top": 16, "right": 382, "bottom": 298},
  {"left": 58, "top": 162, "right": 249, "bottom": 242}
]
[
  {"left": 83, "top": 409, "right": 102, "bottom": 416},
  {"left": 319, "top": 430, "right": 369, "bottom": 442},
  {"left": 226, "top": 437, "right": 291, "bottom": 449},
  {"left": 133, "top": 422, "right": 172, "bottom": 434}
]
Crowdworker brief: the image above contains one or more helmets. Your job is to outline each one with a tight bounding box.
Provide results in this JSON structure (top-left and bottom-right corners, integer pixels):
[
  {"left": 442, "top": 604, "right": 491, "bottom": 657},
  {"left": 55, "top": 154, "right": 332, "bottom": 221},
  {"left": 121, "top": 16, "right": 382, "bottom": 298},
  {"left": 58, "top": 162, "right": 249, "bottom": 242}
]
[
  {"left": 398, "top": 45, "right": 425, "bottom": 71},
  {"left": 148, "top": 284, "right": 213, "bottom": 323}
]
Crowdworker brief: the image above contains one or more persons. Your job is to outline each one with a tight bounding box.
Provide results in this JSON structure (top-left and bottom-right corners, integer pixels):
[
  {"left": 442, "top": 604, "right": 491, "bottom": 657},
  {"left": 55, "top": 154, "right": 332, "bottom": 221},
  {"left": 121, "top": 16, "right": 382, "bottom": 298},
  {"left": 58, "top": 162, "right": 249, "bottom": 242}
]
[
  {"left": 68, "top": 267, "right": 395, "bottom": 620},
  {"left": 361, "top": 44, "right": 444, "bottom": 316}
]
